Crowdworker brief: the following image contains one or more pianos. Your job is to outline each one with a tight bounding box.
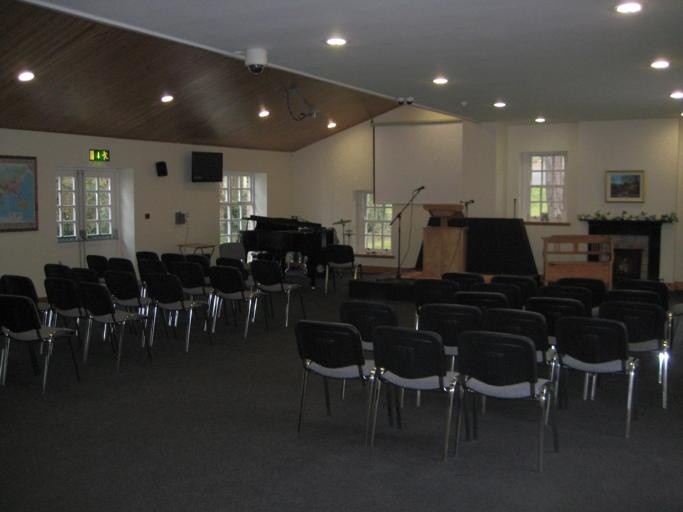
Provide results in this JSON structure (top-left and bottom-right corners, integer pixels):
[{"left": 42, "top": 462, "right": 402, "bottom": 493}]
[{"left": 239, "top": 215, "right": 337, "bottom": 290}]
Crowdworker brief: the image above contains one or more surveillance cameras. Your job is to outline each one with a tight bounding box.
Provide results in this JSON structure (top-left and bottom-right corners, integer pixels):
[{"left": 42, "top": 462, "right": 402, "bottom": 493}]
[{"left": 245, "top": 48, "right": 266, "bottom": 75}]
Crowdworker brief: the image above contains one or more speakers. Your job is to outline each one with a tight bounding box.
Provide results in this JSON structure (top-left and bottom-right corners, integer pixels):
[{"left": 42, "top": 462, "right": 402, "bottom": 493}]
[{"left": 156, "top": 161, "right": 168, "bottom": 176}]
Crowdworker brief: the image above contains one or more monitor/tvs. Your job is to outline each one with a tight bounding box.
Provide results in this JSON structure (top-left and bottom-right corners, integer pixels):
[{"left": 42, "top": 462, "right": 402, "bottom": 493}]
[{"left": 191, "top": 151, "right": 224, "bottom": 182}]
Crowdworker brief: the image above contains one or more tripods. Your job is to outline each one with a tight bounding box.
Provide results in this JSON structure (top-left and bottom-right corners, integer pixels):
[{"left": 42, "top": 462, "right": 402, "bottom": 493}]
[{"left": 376, "top": 192, "right": 419, "bottom": 281}]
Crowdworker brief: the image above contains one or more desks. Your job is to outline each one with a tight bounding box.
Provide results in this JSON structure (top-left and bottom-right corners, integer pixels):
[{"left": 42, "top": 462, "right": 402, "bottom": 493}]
[{"left": 178, "top": 238, "right": 217, "bottom": 256}]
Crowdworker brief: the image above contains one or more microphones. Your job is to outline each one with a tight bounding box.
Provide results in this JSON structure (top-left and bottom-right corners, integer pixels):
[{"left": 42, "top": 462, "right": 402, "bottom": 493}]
[
  {"left": 415, "top": 186, "right": 424, "bottom": 191},
  {"left": 460, "top": 200, "right": 474, "bottom": 204}
]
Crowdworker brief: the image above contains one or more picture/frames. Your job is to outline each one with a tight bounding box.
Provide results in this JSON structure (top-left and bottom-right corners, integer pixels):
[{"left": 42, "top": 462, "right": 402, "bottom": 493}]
[
  {"left": 603, "top": 168, "right": 647, "bottom": 204},
  {"left": 0, "top": 153, "right": 39, "bottom": 234}
]
